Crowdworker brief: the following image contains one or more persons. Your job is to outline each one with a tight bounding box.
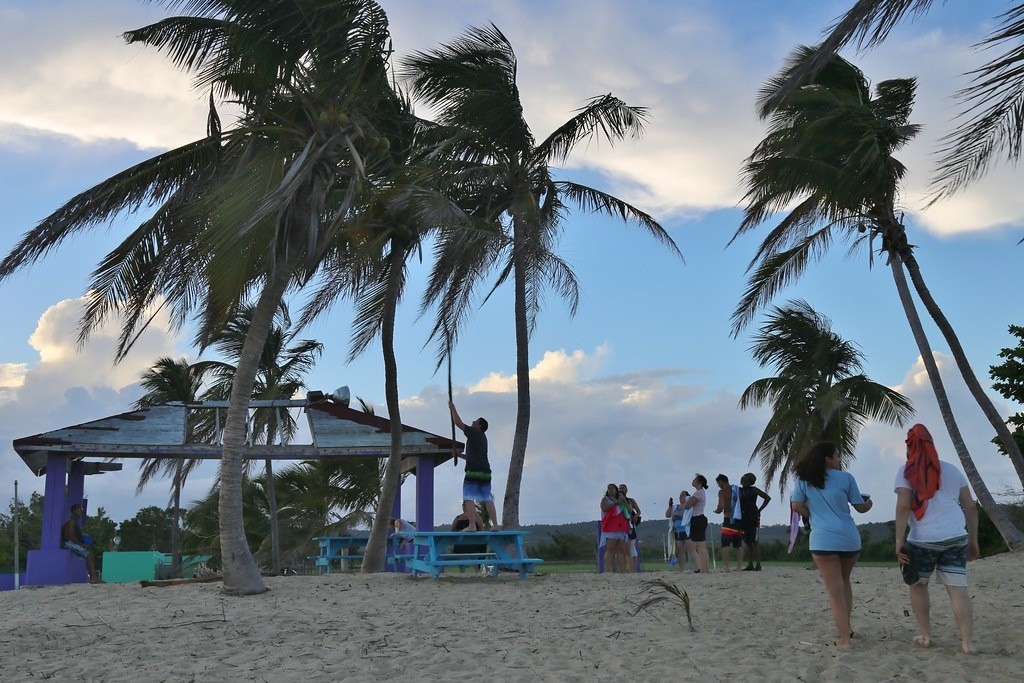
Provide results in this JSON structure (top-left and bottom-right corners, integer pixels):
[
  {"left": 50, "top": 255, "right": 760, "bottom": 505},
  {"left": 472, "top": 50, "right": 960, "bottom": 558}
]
[
  {"left": 448, "top": 401, "right": 500, "bottom": 532},
  {"left": 713, "top": 473, "right": 771, "bottom": 573},
  {"left": 895, "top": 423, "right": 979, "bottom": 656},
  {"left": 61, "top": 504, "right": 107, "bottom": 584},
  {"left": 665, "top": 474, "right": 709, "bottom": 575},
  {"left": 791, "top": 442, "right": 873, "bottom": 650},
  {"left": 600, "top": 484, "right": 641, "bottom": 576},
  {"left": 388, "top": 518, "right": 416, "bottom": 547},
  {"left": 451, "top": 500, "right": 487, "bottom": 570}
]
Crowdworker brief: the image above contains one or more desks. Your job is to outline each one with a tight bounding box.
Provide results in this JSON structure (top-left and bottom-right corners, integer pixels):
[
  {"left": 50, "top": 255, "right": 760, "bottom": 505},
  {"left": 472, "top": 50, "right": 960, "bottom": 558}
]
[
  {"left": 401, "top": 531, "right": 534, "bottom": 582},
  {"left": 312, "top": 536, "right": 401, "bottom": 575}
]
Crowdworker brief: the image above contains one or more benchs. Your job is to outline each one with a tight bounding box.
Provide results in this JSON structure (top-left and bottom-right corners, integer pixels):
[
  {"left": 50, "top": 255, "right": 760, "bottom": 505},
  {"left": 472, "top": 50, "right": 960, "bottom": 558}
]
[
  {"left": 395, "top": 553, "right": 496, "bottom": 559},
  {"left": 307, "top": 555, "right": 395, "bottom": 560},
  {"left": 424, "top": 559, "right": 545, "bottom": 565}
]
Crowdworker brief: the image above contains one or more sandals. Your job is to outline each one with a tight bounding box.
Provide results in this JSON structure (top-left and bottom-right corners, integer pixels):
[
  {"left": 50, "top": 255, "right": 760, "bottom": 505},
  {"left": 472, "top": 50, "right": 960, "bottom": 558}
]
[
  {"left": 848, "top": 628, "right": 854, "bottom": 638},
  {"left": 825, "top": 640, "right": 838, "bottom": 647}
]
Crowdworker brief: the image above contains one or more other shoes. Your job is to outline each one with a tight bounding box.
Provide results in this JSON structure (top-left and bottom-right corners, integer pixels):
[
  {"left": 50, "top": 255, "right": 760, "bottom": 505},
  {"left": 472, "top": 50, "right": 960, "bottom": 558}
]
[
  {"left": 742, "top": 565, "right": 754, "bottom": 571},
  {"left": 900, "top": 545, "right": 921, "bottom": 588},
  {"left": 694, "top": 568, "right": 701, "bottom": 573},
  {"left": 752, "top": 566, "right": 761, "bottom": 571}
]
